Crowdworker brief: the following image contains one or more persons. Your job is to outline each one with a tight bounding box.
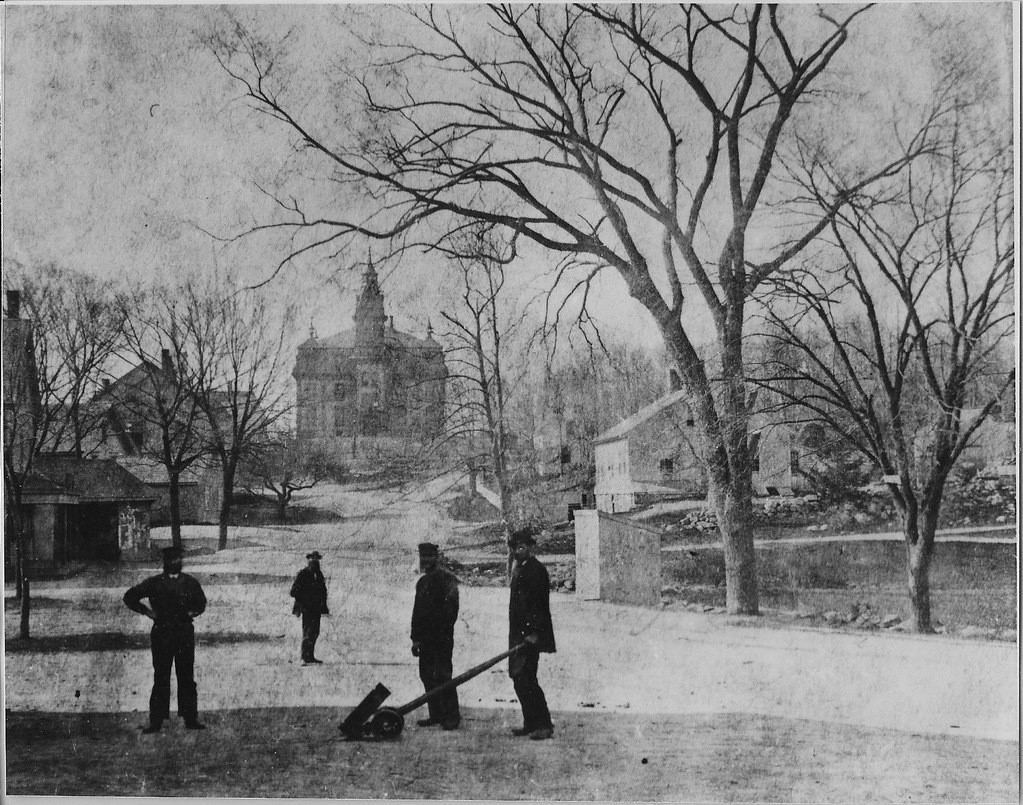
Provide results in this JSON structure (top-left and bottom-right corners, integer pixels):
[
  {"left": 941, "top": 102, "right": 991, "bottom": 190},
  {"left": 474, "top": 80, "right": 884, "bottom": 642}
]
[
  {"left": 504, "top": 531, "right": 558, "bottom": 740},
  {"left": 408, "top": 542, "right": 465, "bottom": 730},
  {"left": 288, "top": 549, "right": 332, "bottom": 666},
  {"left": 121, "top": 547, "right": 212, "bottom": 733}
]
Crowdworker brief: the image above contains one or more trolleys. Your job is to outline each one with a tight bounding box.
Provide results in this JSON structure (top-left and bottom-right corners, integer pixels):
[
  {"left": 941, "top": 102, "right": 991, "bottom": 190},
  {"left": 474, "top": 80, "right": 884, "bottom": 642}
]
[{"left": 339, "top": 643, "right": 518, "bottom": 737}]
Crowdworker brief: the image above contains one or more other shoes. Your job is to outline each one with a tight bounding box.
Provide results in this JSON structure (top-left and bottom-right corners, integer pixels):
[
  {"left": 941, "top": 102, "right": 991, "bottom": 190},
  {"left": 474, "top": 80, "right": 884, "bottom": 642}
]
[
  {"left": 443, "top": 718, "right": 460, "bottom": 729},
  {"left": 417, "top": 717, "right": 441, "bottom": 726},
  {"left": 186, "top": 722, "right": 205, "bottom": 729},
  {"left": 512, "top": 725, "right": 534, "bottom": 736},
  {"left": 142, "top": 724, "right": 160, "bottom": 733},
  {"left": 304, "top": 656, "right": 322, "bottom": 663},
  {"left": 530, "top": 728, "right": 554, "bottom": 740}
]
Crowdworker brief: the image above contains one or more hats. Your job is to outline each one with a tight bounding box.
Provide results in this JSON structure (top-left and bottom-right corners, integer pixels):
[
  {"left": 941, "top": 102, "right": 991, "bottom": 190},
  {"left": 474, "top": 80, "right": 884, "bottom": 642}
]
[
  {"left": 507, "top": 530, "right": 536, "bottom": 546},
  {"left": 163, "top": 547, "right": 184, "bottom": 557},
  {"left": 306, "top": 551, "right": 322, "bottom": 559},
  {"left": 418, "top": 543, "right": 439, "bottom": 555}
]
